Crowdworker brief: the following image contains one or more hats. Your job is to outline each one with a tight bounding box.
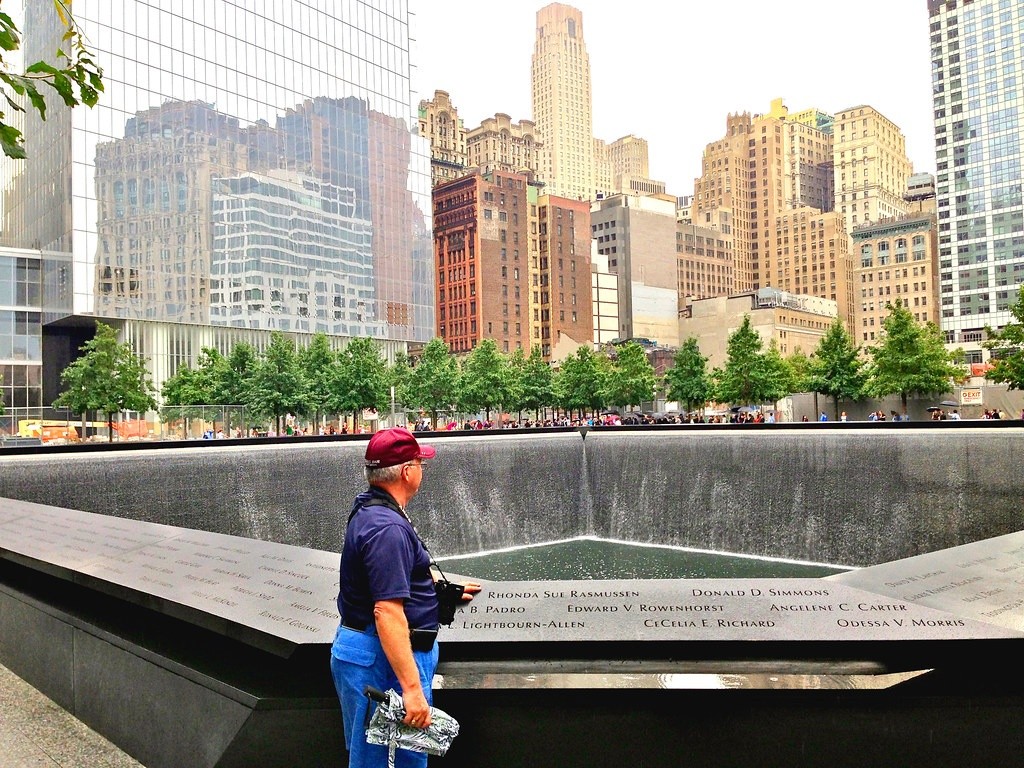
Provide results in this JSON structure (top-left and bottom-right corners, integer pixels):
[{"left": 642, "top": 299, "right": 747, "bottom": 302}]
[{"left": 365, "top": 427, "right": 436, "bottom": 469}]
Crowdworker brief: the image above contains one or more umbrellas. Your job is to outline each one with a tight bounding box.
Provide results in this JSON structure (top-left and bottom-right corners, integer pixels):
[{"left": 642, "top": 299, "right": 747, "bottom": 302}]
[
  {"left": 361, "top": 685, "right": 460, "bottom": 768},
  {"left": 249, "top": 424, "right": 262, "bottom": 429},
  {"left": 927, "top": 407, "right": 941, "bottom": 413},
  {"left": 890, "top": 410, "right": 901, "bottom": 418},
  {"left": 601, "top": 406, "right": 779, "bottom": 419},
  {"left": 940, "top": 401, "right": 959, "bottom": 407}
]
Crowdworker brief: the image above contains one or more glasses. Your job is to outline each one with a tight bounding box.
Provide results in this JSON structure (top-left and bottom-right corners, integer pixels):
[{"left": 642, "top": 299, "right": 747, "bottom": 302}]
[{"left": 400, "top": 461, "right": 427, "bottom": 476}]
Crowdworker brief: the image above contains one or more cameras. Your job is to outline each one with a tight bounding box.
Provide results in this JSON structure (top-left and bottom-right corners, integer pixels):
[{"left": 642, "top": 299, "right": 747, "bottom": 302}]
[{"left": 435, "top": 580, "right": 465, "bottom": 625}]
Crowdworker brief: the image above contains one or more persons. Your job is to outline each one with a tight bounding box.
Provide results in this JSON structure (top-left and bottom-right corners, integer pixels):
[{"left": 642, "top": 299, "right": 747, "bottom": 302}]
[
  {"left": 202, "top": 421, "right": 433, "bottom": 440},
  {"left": 331, "top": 426, "right": 482, "bottom": 768},
  {"left": 445, "top": 413, "right": 775, "bottom": 430},
  {"left": 802, "top": 409, "right": 1024, "bottom": 422}
]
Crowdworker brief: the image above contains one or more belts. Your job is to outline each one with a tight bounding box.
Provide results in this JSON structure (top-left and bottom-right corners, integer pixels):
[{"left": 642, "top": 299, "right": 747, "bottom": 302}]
[{"left": 341, "top": 618, "right": 378, "bottom": 634}]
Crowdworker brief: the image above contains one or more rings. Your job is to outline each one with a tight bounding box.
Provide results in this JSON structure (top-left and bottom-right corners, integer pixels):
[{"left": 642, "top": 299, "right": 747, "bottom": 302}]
[{"left": 410, "top": 720, "right": 417, "bottom": 727}]
